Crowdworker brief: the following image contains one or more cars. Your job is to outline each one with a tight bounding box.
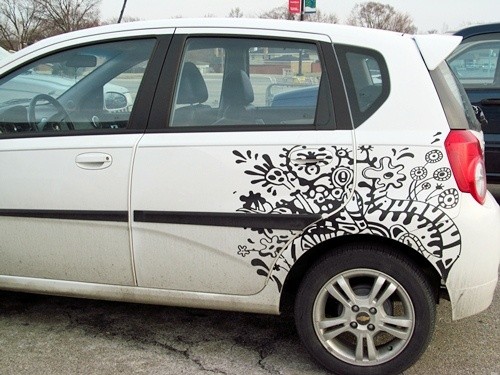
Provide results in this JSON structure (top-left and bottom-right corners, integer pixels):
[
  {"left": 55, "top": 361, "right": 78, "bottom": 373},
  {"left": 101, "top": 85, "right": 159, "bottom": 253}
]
[
  {"left": 273, "top": 22, "right": 500, "bottom": 200},
  {"left": 0, "top": 17, "right": 500, "bottom": 375},
  {"left": 0, "top": 44, "right": 132, "bottom": 129}
]
